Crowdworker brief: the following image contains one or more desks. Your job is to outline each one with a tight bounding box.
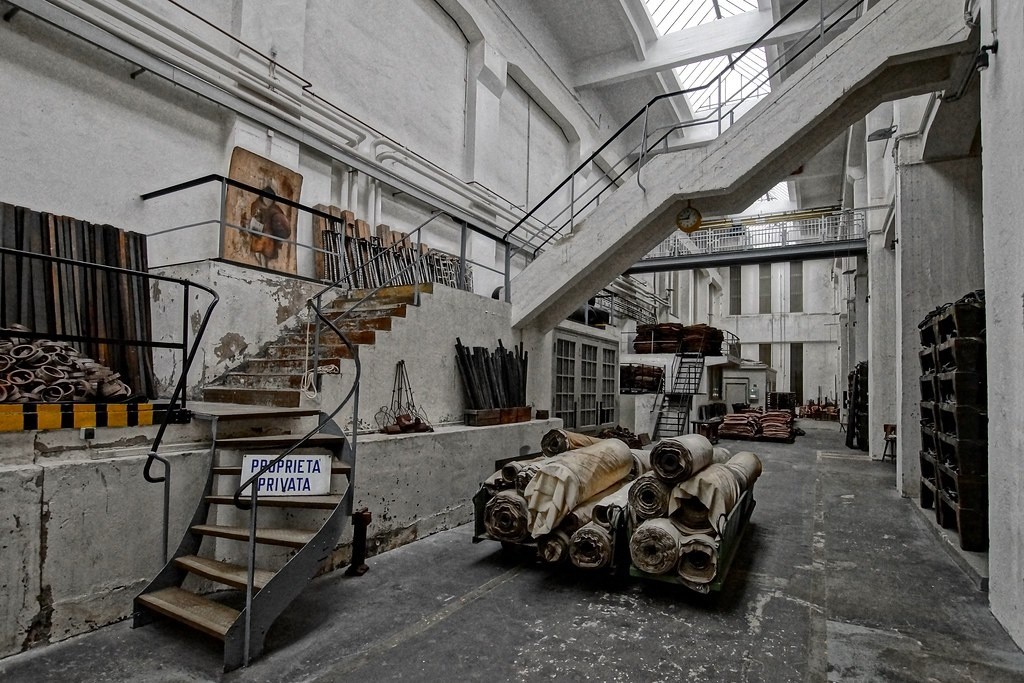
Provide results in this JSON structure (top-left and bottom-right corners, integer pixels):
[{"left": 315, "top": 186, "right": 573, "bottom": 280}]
[{"left": 691, "top": 420, "right": 719, "bottom": 444}]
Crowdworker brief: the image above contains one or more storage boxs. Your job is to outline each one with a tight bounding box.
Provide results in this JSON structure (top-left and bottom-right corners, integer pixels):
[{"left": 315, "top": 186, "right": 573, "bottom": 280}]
[
  {"left": 464, "top": 408, "right": 500, "bottom": 426},
  {"left": 515, "top": 407, "right": 532, "bottom": 421},
  {"left": 500, "top": 408, "right": 517, "bottom": 423}
]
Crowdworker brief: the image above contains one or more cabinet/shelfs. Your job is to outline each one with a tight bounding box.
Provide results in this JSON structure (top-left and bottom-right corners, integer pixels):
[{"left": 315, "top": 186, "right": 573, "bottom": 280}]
[
  {"left": 847, "top": 364, "right": 868, "bottom": 451},
  {"left": 919, "top": 303, "right": 989, "bottom": 552}
]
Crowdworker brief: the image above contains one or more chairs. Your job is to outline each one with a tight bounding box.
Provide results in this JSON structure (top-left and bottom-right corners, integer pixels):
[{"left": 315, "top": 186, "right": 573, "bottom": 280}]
[
  {"left": 798, "top": 405, "right": 838, "bottom": 420},
  {"left": 882, "top": 424, "right": 896, "bottom": 464}
]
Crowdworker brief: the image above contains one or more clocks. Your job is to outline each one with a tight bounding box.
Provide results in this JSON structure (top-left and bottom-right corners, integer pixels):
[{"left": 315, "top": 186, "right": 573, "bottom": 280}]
[{"left": 675, "top": 206, "right": 701, "bottom": 233}]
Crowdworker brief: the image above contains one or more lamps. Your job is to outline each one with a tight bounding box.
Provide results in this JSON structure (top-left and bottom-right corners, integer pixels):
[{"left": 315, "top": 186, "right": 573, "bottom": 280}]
[
  {"left": 867, "top": 123, "right": 897, "bottom": 141},
  {"left": 852, "top": 320, "right": 857, "bottom": 327},
  {"left": 838, "top": 346, "right": 840, "bottom": 350},
  {"left": 845, "top": 322, "right": 848, "bottom": 327},
  {"left": 864, "top": 295, "right": 870, "bottom": 303},
  {"left": 975, "top": 38, "right": 998, "bottom": 71},
  {"left": 890, "top": 237, "right": 898, "bottom": 250}
]
